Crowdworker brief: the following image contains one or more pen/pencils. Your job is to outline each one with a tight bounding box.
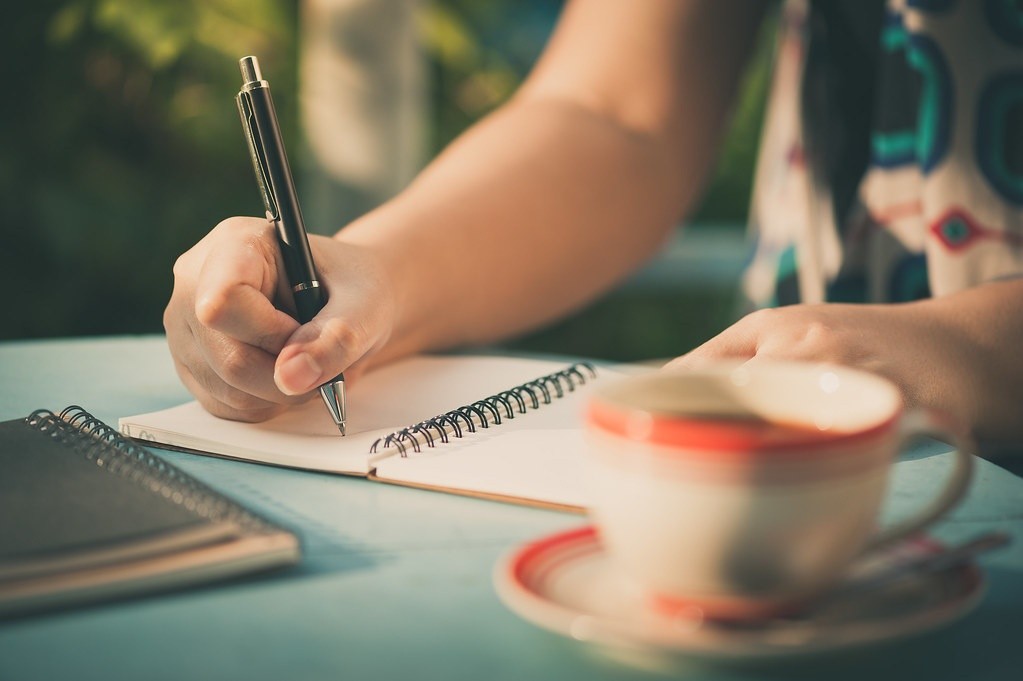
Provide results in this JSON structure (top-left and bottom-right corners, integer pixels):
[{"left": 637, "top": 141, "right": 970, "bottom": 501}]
[{"left": 235, "top": 56, "right": 347, "bottom": 436}]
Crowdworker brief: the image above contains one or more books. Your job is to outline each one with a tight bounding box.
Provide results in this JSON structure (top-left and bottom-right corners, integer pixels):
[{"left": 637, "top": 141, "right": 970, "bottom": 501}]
[
  {"left": 122, "top": 358, "right": 646, "bottom": 519},
  {"left": 0, "top": 405, "right": 307, "bottom": 623}
]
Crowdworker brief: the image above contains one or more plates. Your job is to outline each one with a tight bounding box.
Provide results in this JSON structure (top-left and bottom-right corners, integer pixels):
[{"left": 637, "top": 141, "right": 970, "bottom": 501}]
[{"left": 492, "top": 522, "right": 988, "bottom": 674}]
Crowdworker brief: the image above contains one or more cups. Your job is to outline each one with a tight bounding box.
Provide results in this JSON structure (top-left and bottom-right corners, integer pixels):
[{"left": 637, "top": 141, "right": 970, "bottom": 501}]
[{"left": 581, "top": 359, "right": 973, "bottom": 620}]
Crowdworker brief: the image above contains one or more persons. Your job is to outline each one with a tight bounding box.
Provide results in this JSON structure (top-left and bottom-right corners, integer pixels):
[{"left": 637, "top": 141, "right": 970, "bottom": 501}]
[{"left": 161, "top": 0, "right": 1023, "bottom": 462}]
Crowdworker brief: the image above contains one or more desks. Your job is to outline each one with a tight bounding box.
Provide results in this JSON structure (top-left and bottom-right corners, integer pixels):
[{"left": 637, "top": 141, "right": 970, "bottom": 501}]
[{"left": 0, "top": 336, "right": 1023, "bottom": 681}]
